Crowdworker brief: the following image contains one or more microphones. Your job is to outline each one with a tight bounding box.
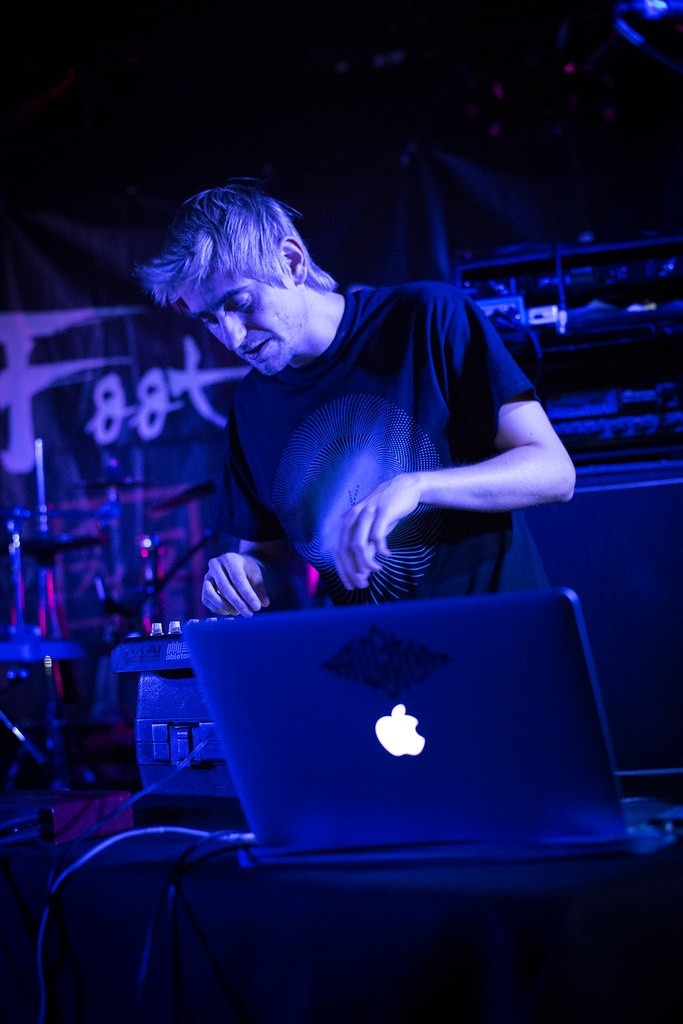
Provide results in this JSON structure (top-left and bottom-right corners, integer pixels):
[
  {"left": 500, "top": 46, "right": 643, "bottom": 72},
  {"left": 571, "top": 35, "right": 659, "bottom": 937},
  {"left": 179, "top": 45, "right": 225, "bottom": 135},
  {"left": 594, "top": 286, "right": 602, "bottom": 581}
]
[
  {"left": 633, "top": 0, "right": 683, "bottom": 20},
  {"left": 97, "top": 576, "right": 119, "bottom": 644},
  {"left": 144, "top": 478, "right": 217, "bottom": 517}
]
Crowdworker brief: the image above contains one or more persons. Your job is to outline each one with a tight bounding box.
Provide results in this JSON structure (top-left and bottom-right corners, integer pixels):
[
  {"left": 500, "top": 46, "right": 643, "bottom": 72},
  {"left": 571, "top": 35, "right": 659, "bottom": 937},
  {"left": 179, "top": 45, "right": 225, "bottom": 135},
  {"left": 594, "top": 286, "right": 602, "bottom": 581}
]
[{"left": 140, "top": 184, "right": 576, "bottom": 616}]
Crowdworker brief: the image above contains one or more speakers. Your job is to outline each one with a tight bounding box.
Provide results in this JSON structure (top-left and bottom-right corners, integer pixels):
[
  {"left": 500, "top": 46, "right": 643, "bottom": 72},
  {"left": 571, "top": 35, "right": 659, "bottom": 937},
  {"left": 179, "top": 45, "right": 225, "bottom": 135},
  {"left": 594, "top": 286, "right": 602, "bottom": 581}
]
[{"left": 520, "top": 462, "right": 683, "bottom": 772}]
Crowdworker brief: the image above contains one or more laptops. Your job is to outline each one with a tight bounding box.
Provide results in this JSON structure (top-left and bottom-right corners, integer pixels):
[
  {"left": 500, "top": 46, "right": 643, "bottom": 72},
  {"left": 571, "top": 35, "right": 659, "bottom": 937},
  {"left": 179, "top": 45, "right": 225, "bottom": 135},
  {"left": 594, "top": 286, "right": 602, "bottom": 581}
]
[{"left": 189, "top": 588, "right": 683, "bottom": 859}]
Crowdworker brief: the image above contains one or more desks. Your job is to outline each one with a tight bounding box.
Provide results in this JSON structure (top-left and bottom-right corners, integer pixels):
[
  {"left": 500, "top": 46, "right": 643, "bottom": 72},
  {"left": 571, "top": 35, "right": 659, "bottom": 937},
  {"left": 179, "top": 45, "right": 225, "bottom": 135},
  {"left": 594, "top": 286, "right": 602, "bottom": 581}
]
[{"left": 0, "top": 792, "right": 683, "bottom": 1024}]
